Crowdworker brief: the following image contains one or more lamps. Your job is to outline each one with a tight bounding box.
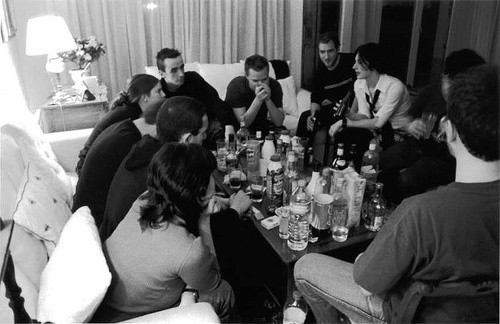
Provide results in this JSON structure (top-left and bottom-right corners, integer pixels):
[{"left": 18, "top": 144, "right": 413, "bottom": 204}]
[{"left": 25, "top": 16, "right": 77, "bottom": 99}]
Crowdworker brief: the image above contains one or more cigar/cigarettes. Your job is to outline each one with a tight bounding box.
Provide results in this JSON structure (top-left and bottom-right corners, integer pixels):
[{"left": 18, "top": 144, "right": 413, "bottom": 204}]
[{"left": 245, "top": 191, "right": 253, "bottom": 195}]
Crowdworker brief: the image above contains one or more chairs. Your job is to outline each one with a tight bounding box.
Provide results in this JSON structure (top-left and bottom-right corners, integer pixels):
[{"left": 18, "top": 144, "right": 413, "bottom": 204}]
[{"left": 395, "top": 280, "right": 499, "bottom": 323}]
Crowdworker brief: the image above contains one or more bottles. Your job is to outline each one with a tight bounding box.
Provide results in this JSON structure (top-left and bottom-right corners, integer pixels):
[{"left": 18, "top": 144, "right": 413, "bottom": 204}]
[
  {"left": 363, "top": 182, "right": 386, "bottom": 232},
  {"left": 320, "top": 168, "right": 332, "bottom": 195},
  {"left": 331, "top": 178, "right": 350, "bottom": 242},
  {"left": 286, "top": 179, "right": 312, "bottom": 251},
  {"left": 335, "top": 90, "right": 352, "bottom": 117},
  {"left": 266, "top": 155, "right": 284, "bottom": 201},
  {"left": 360, "top": 143, "right": 380, "bottom": 182},
  {"left": 236, "top": 116, "right": 250, "bottom": 143},
  {"left": 348, "top": 143, "right": 359, "bottom": 172},
  {"left": 282, "top": 291, "right": 309, "bottom": 324},
  {"left": 226, "top": 133, "right": 239, "bottom": 169},
  {"left": 261, "top": 135, "right": 276, "bottom": 160},
  {"left": 275, "top": 139, "right": 286, "bottom": 161},
  {"left": 268, "top": 126, "right": 277, "bottom": 146},
  {"left": 305, "top": 172, "right": 324, "bottom": 194},
  {"left": 278, "top": 214, "right": 290, "bottom": 239},
  {"left": 278, "top": 129, "right": 291, "bottom": 144},
  {"left": 329, "top": 143, "right": 347, "bottom": 170},
  {"left": 283, "top": 151, "right": 301, "bottom": 195},
  {"left": 310, "top": 110, "right": 319, "bottom": 135},
  {"left": 255, "top": 131, "right": 264, "bottom": 152}
]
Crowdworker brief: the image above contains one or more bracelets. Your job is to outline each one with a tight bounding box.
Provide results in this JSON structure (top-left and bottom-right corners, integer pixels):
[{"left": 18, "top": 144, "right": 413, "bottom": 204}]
[{"left": 342, "top": 117, "right": 347, "bottom": 127}]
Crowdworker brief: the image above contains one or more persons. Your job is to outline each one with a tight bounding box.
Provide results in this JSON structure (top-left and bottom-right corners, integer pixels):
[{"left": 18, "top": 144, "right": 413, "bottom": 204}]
[
  {"left": 381, "top": 48, "right": 487, "bottom": 204},
  {"left": 328, "top": 42, "right": 412, "bottom": 176},
  {"left": 296, "top": 35, "right": 355, "bottom": 166},
  {"left": 102, "top": 142, "right": 235, "bottom": 324},
  {"left": 75, "top": 73, "right": 167, "bottom": 178},
  {"left": 70, "top": 99, "right": 168, "bottom": 229},
  {"left": 101, "top": 97, "right": 254, "bottom": 242},
  {"left": 294, "top": 62, "right": 500, "bottom": 324},
  {"left": 156, "top": 47, "right": 240, "bottom": 149},
  {"left": 224, "top": 54, "right": 285, "bottom": 139}
]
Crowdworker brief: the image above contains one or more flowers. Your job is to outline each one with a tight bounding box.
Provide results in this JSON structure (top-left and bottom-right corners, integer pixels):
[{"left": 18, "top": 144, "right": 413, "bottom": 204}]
[{"left": 49, "top": 35, "right": 107, "bottom": 70}]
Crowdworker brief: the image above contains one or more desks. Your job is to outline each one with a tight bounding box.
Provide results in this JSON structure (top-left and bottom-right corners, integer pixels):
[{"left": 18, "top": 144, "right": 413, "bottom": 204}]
[{"left": 44, "top": 81, "right": 107, "bottom": 132}]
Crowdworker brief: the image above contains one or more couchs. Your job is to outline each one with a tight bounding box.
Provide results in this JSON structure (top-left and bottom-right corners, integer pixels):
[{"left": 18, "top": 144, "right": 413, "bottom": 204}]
[
  {"left": 0, "top": 110, "right": 221, "bottom": 324},
  {"left": 145, "top": 60, "right": 312, "bottom": 137}
]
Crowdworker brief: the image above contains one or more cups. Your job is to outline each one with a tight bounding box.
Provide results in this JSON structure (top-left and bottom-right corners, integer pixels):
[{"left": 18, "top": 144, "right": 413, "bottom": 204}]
[
  {"left": 228, "top": 164, "right": 241, "bottom": 189},
  {"left": 215, "top": 139, "right": 227, "bottom": 173},
  {"left": 312, "top": 193, "right": 334, "bottom": 231},
  {"left": 249, "top": 175, "right": 264, "bottom": 203},
  {"left": 421, "top": 112, "right": 437, "bottom": 140}
]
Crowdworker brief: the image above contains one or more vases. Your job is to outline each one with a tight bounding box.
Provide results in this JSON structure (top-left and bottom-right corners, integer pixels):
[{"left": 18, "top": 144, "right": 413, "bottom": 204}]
[{"left": 68, "top": 69, "right": 92, "bottom": 90}]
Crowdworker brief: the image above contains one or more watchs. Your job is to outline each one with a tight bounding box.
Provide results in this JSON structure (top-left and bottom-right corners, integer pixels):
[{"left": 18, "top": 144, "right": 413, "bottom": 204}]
[{"left": 183, "top": 288, "right": 199, "bottom": 299}]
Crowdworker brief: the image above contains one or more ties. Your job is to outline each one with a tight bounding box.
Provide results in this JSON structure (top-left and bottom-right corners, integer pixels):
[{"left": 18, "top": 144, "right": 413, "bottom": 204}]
[{"left": 365, "top": 90, "right": 379, "bottom": 117}]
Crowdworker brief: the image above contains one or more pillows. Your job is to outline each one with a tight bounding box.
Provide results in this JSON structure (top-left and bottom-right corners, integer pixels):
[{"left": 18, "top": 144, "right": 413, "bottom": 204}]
[
  {"left": 12, "top": 161, "right": 73, "bottom": 259},
  {"left": 36, "top": 206, "right": 113, "bottom": 324},
  {"left": 277, "top": 76, "right": 298, "bottom": 117}
]
